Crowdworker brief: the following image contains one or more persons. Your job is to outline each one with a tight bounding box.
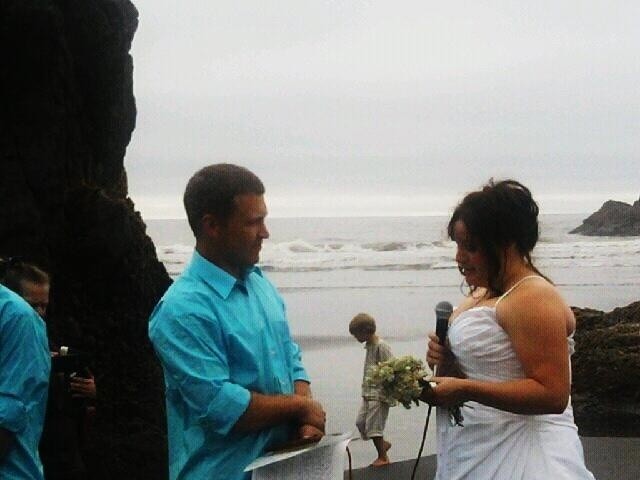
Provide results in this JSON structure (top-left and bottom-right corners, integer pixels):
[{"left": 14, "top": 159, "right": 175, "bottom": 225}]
[
  {"left": 0, "top": 257, "right": 105, "bottom": 480},
  {"left": 347, "top": 312, "right": 399, "bottom": 467},
  {"left": 145, "top": 161, "right": 327, "bottom": 480},
  {"left": 417, "top": 179, "right": 597, "bottom": 480}
]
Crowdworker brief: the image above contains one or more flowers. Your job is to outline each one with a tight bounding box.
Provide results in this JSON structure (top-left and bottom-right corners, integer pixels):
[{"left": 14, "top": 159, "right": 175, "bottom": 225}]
[{"left": 361, "top": 354, "right": 476, "bottom": 427}]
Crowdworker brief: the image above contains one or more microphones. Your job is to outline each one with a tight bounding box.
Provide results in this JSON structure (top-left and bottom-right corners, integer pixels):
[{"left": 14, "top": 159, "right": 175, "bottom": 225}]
[{"left": 430, "top": 301, "right": 454, "bottom": 364}]
